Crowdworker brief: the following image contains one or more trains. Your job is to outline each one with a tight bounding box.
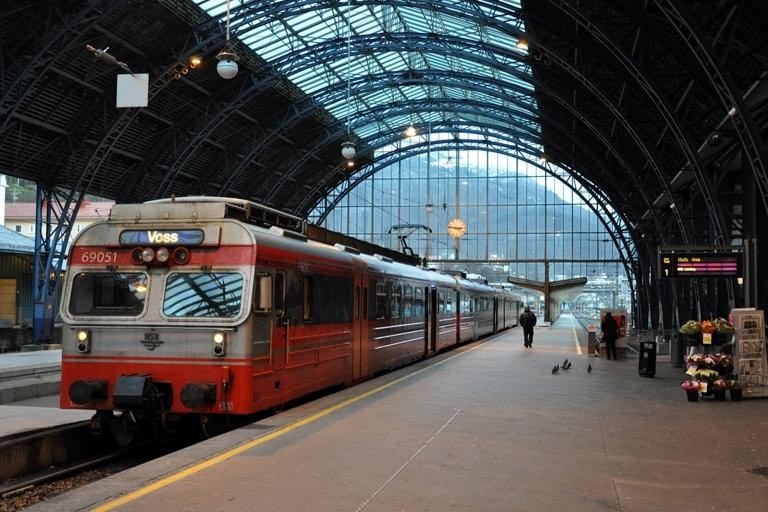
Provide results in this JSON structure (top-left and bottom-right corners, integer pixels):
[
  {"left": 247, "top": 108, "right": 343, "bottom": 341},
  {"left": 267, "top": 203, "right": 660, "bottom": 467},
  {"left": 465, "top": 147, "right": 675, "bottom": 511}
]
[{"left": 56, "top": 189, "right": 523, "bottom": 441}]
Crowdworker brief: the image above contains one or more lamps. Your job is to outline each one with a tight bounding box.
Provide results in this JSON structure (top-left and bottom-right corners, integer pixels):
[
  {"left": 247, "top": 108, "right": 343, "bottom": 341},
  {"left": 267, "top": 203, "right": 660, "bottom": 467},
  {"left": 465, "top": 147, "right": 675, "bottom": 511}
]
[
  {"left": 340, "top": 2, "right": 357, "bottom": 159},
  {"left": 214, "top": 1, "right": 240, "bottom": 79},
  {"left": 403, "top": 71, "right": 416, "bottom": 138},
  {"left": 424, "top": 73, "right": 435, "bottom": 214}
]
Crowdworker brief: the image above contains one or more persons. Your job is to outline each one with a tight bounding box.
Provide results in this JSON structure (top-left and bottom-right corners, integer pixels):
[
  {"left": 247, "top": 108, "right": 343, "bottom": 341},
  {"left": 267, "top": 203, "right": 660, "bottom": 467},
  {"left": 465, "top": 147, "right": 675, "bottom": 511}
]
[
  {"left": 601, "top": 311, "right": 619, "bottom": 360},
  {"left": 519, "top": 305, "right": 536, "bottom": 348}
]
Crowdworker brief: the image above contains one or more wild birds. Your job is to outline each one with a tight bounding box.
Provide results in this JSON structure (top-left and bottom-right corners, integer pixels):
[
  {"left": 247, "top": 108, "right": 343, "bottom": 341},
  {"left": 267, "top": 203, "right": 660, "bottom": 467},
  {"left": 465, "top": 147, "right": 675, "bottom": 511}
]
[
  {"left": 567, "top": 362, "right": 571, "bottom": 369},
  {"left": 551, "top": 366, "right": 556, "bottom": 374},
  {"left": 587, "top": 363, "right": 592, "bottom": 374},
  {"left": 556, "top": 364, "right": 559, "bottom": 372},
  {"left": 560, "top": 358, "right": 568, "bottom": 369}
]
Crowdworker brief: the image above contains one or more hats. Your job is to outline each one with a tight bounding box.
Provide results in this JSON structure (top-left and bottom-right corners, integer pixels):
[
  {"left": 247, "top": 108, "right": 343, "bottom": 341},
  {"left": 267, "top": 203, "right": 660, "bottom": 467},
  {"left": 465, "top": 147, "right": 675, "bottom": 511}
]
[{"left": 523, "top": 305, "right": 530, "bottom": 313}]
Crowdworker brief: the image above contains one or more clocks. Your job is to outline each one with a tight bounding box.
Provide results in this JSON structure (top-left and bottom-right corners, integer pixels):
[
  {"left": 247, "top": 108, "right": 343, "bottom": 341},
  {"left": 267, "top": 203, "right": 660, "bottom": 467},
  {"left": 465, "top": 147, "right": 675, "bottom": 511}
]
[{"left": 446, "top": 218, "right": 466, "bottom": 239}]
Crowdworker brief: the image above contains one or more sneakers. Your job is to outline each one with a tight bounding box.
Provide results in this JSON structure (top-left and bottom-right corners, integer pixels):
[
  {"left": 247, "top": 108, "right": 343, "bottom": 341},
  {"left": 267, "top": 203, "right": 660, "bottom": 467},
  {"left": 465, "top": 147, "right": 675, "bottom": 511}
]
[
  {"left": 604, "top": 354, "right": 618, "bottom": 360},
  {"left": 524, "top": 342, "right": 532, "bottom": 348}
]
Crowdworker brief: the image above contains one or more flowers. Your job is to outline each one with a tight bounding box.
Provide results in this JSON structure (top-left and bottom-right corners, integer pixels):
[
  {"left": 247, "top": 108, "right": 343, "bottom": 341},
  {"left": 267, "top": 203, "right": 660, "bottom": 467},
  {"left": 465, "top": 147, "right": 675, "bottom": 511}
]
[{"left": 679, "top": 319, "right": 744, "bottom": 403}]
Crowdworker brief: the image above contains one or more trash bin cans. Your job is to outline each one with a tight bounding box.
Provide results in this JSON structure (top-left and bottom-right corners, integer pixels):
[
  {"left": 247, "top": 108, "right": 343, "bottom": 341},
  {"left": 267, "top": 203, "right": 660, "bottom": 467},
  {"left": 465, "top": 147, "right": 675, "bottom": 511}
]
[{"left": 638, "top": 340, "right": 657, "bottom": 377}]
